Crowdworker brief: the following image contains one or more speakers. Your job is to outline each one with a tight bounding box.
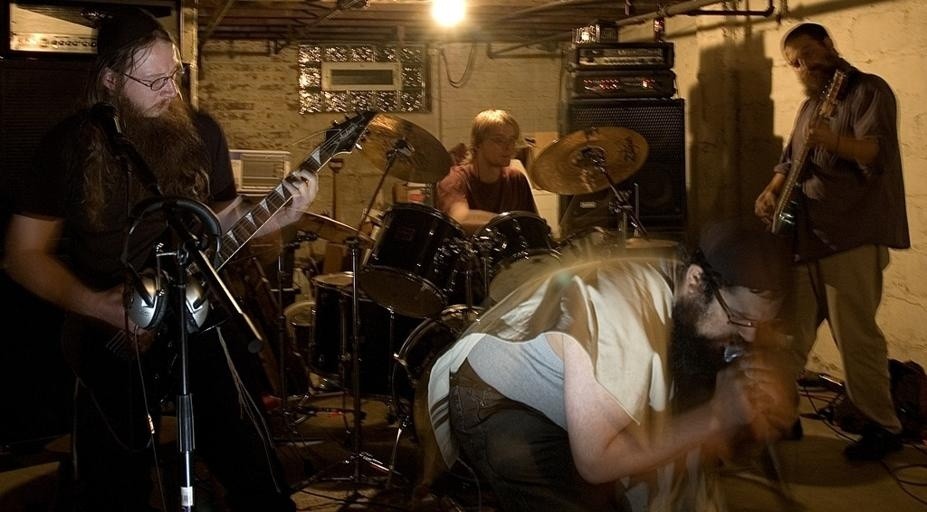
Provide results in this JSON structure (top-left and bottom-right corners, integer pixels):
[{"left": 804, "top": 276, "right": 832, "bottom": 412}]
[{"left": 557, "top": 98, "right": 687, "bottom": 232}]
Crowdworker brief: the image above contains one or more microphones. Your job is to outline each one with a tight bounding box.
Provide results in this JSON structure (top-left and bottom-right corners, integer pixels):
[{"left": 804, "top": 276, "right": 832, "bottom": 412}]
[
  {"left": 90, "top": 99, "right": 126, "bottom": 154},
  {"left": 723, "top": 343, "right": 780, "bottom": 475},
  {"left": 131, "top": 324, "right": 157, "bottom": 436}
]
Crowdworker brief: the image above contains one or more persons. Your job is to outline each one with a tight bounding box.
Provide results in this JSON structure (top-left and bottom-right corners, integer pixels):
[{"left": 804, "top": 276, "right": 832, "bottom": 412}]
[
  {"left": 448, "top": 230, "right": 788, "bottom": 512},
  {"left": 3, "top": 9, "right": 318, "bottom": 512},
  {"left": 436, "top": 110, "right": 539, "bottom": 232},
  {"left": 754, "top": 23, "right": 909, "bottom": 463}
]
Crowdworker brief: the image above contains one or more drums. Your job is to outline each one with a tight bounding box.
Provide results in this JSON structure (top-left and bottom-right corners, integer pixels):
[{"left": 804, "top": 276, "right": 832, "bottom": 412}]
[
  {"left": 312, "top": 270, "right": 359, "bottom": 390},
  {"left": 284, "top": 300, "right": 319, "bottom": 368},
  {"left": 357, "top": 202, "right": 474, "bottom": 319},
  {"left": 391, "top": 304, "right": 489, "bottom": 470},
  {"left": 338, "top": 285, "right": 425, "bottom": 405},
  {"left": 470, "top": 211, "right": 565, "bottom": 304}
]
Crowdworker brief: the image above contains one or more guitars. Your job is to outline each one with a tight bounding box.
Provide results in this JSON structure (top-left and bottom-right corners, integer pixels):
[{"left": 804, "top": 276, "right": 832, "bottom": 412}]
[
  {"left": 60, "top": 109, "right": 378, "bottom": 450},
  {"left": 770, "top": 70, "right": 847, "bottom": 236}
]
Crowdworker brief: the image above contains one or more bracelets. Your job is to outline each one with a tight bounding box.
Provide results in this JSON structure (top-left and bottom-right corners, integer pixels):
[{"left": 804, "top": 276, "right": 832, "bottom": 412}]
[{"left": 836, "top": 135, "right": 840, "bottom": 149}]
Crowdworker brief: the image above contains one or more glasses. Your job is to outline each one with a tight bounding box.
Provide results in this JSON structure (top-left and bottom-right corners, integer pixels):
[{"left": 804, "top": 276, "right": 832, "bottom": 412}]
[
  {"left": 699, "top": 263, "right": 764, "bottom": 330},
  {"left": 108, "top": 65, "right": 180, "bottom": 91}
]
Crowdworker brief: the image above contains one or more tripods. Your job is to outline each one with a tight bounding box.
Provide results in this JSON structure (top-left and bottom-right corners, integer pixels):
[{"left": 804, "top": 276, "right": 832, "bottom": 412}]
[{"left": 266, "top": 241, "right": 418, "bottom": 512}]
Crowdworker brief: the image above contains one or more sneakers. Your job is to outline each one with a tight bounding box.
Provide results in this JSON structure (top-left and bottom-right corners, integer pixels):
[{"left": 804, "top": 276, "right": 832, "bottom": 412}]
[
  {"left": 783, "top": 418, "right": 802, "bottom": 440},
  {"left": 845, "top": 436, "right": 904, "bottom": 463}
]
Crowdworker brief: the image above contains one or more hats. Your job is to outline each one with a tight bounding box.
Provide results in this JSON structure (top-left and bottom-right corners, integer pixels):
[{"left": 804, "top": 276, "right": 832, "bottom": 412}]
[
  {"left": 707, "top": 236, "right": 793, "bottom": 289},
  {"left": 97, "top": 8, "right": 159, "bottom": 50}
]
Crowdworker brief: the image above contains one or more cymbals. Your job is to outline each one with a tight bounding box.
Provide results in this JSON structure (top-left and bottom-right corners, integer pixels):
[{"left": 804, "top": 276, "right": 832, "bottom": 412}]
[
  {"left": 278, "top": 207, "right": 375, "bottom": 251},
  {"left": 530, "top": 126, "right": 649, "bottom": 195},
  {"left": 623, "top": 238, "right": 678, "bottom": 249},
  {"left": 354, "top": 113, "right": 451, "bottom": 183}
]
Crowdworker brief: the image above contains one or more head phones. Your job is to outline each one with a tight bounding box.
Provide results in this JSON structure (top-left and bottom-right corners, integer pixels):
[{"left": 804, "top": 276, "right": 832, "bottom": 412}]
[{"left": 117, "top": 193, "right": 223, "bottom": 337}]
